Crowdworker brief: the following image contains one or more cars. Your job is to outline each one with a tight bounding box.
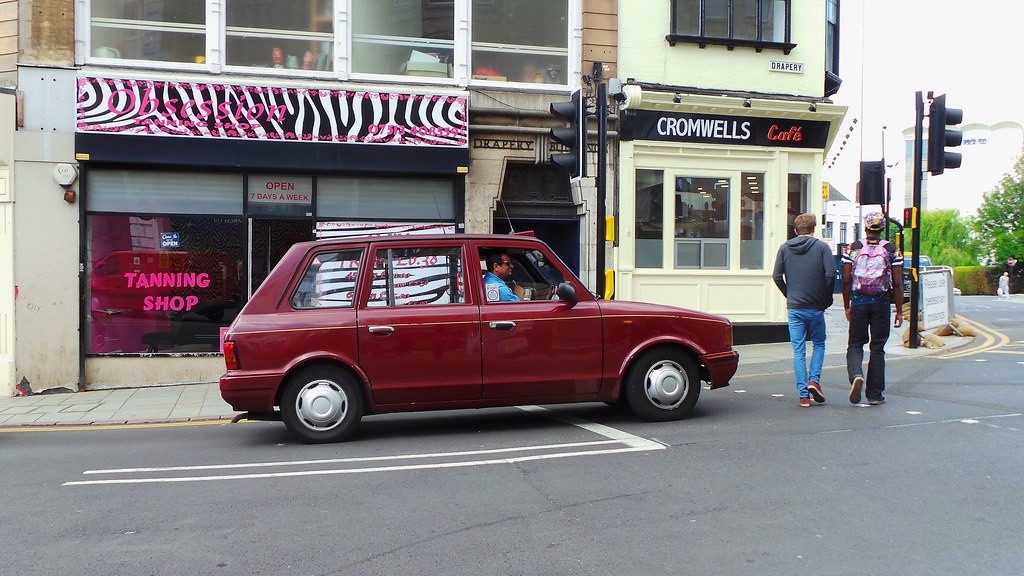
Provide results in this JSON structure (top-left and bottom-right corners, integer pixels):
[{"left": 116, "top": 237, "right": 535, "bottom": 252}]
[
  {"left": 217, "top": 231, "right": 742, "bottom": 444},
  {"left": 953, "top": 287, "right": 962, "bottom": 296}
]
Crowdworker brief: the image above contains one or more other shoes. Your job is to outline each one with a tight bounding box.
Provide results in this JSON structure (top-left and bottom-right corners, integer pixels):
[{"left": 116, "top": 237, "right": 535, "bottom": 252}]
[
  {"left": 807, "top": 382, "right": 825, "bottom": 403},
  {"left": 867, "top": 396, "right": 886, "bottom": 404},
  {"left": 799, "top": 398, "right": 810, "bottom": 407},
  {"left": 849, "top": 376, "right": 864, "bottom": 404}
]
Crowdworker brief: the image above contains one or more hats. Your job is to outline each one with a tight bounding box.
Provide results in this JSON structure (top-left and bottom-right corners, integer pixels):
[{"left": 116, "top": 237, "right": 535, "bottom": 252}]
[{"left": 864, "top": 211, "right": 884, "bottom": 230}]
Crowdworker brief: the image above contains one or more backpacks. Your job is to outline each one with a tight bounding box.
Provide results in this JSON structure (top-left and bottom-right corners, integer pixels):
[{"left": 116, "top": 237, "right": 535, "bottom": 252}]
[{"left": 851, "top": 238, "right": 891, "bottom": 295}]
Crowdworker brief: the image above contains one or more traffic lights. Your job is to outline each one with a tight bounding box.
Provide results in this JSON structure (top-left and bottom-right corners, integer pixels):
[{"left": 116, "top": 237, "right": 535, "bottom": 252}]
[
  {"left": 902, "top": 207, "right": 917, "bottom": 230},
  {"left": 928, "top": 93, "right": 966, "bottom": 177},
  {"left": 547, "top": 86, "right": 584, "bottom": 178}
]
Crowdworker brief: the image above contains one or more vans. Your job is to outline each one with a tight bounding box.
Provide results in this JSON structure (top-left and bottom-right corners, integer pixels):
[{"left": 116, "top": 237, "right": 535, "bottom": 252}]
[{"left": 891, "top": 254, "right": 933, "bottom": 302}]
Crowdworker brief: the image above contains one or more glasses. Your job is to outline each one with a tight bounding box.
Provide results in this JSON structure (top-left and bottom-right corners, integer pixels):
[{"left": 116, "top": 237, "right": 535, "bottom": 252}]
[{"left": 496, "top": 260, "right": 513, "bottom": 267}]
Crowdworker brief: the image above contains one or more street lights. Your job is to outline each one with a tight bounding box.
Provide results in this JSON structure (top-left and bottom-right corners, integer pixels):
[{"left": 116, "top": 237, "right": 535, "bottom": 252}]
[{"left": 880, "top": 125, "right": 890, "bottom": 177}]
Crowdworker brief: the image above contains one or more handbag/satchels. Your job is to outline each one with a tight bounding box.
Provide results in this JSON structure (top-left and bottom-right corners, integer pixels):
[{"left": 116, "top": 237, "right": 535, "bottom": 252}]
[
  {"left": 997, "top": 288, "right": 1003, "bottom": 296},
  {"left": 1007, "top": 297, "right": 1012, "bottom": 300},
  {"left": 998, "top": 297, "right": 1001, "bottom": 300}
]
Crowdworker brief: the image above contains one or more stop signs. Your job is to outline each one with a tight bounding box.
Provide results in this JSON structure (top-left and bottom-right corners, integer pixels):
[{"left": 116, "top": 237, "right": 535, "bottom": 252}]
[{"left": 1008, "top": 257, "right": 1014, "bottom": 266}]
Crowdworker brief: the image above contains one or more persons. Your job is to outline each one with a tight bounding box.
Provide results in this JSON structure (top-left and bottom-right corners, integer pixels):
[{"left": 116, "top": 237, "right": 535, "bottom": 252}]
[
  {"left": 773, "top": 213, "right": 835, "bottom": 406},
  {"left": 841, "top": 211, "right": 904, "bottom": 404},
  {"left": 483, "top": 250, "right": 525, "bottom": 302},
  {"left": 998, "top": 272, "right": 1011, "bottom": 300}
]
